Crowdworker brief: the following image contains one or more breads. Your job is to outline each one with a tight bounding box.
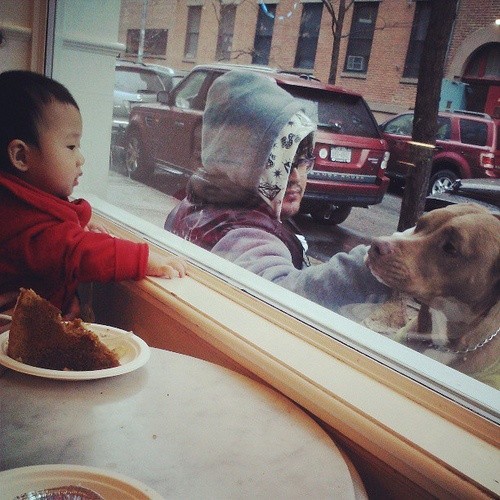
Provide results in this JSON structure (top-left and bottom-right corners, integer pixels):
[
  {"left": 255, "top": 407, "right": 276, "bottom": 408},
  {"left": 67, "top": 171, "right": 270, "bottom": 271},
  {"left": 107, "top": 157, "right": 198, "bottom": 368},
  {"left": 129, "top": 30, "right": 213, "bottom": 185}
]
[{"left": 6, "top": 289, "right": 121, "bottom": 371}]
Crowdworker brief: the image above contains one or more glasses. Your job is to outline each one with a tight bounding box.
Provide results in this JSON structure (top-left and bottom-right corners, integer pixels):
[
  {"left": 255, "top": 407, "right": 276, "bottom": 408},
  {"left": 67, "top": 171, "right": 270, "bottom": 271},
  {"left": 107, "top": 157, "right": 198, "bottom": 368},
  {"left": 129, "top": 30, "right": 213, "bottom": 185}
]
[{"left": 289, "top": 152, "right": 316, "bottom": 175}]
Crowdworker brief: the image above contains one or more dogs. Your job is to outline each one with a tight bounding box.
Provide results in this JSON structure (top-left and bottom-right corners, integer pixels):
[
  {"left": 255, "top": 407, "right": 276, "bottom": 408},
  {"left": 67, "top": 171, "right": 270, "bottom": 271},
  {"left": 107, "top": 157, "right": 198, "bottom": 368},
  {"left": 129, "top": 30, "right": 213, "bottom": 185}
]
[{"left": 367, "top": 201, "right": 499, "bottom": 395}]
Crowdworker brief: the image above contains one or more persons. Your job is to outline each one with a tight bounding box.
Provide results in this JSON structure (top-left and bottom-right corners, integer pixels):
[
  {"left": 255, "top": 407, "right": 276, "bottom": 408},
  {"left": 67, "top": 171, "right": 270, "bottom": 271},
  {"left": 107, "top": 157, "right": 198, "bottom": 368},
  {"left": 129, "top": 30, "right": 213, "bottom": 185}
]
[
  {"left": 164, "top": 68, "right": 417, "bottom": 310},
  {"left": 0, "top": 70, "right": 190, "bottom": 335}
]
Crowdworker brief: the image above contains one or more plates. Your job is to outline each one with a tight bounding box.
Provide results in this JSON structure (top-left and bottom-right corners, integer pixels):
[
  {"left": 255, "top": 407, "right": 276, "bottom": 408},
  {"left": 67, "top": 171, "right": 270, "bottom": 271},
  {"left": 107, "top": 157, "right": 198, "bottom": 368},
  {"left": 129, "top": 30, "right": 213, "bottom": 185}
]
[
  {"left": 0, "top": 322, "right": 151, "bottom": 380},
  {"left": 0, "top": 464, "right": 164, "bottom": 500}
]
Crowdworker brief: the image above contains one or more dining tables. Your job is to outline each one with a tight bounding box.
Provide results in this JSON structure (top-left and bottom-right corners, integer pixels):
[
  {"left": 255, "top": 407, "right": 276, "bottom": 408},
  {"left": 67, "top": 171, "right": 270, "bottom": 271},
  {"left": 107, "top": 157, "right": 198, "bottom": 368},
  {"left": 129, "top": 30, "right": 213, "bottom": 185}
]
[{"left": 3, "top": 347, "right": 367, "bottom": 496}]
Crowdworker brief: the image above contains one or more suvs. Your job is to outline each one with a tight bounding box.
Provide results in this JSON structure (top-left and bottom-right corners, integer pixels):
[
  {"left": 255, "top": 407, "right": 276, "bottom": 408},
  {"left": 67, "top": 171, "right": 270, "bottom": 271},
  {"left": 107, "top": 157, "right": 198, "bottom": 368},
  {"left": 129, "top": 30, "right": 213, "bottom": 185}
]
[
  {"left": 110, "top": 60, "right": 390, "bottom": 226},
  {"left": 377, "top": 109, "right": 500, "bottom": 195}
]
